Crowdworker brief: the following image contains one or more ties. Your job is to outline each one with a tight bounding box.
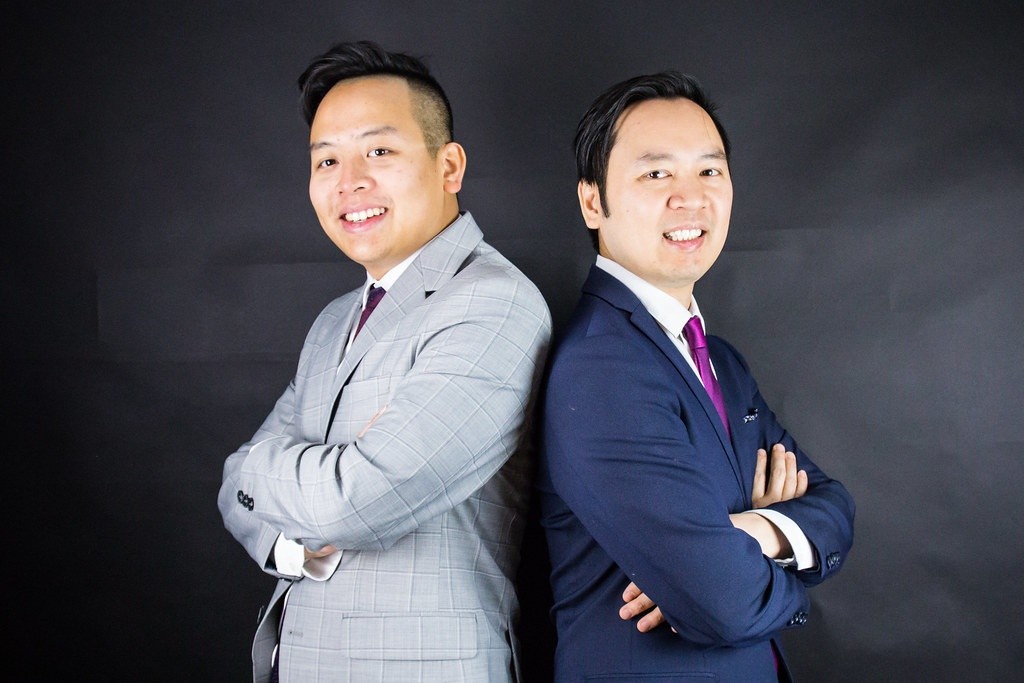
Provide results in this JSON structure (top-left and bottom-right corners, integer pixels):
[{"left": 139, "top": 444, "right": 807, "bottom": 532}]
[
  {"left": 353, "top": 286, "right": 386, "bottom": 341},
  {"left": 682, "top": 315, "right": 732, "bottom": 443}
]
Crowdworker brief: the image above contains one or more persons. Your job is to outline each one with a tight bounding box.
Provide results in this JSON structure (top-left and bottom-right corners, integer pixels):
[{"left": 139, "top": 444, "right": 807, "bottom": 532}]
[
  {"left": 530, "top": 65, "right": 858, "bottom": 683},
  {"left": 215, "top": 37, "right": 558, "bottom": 683}
]
